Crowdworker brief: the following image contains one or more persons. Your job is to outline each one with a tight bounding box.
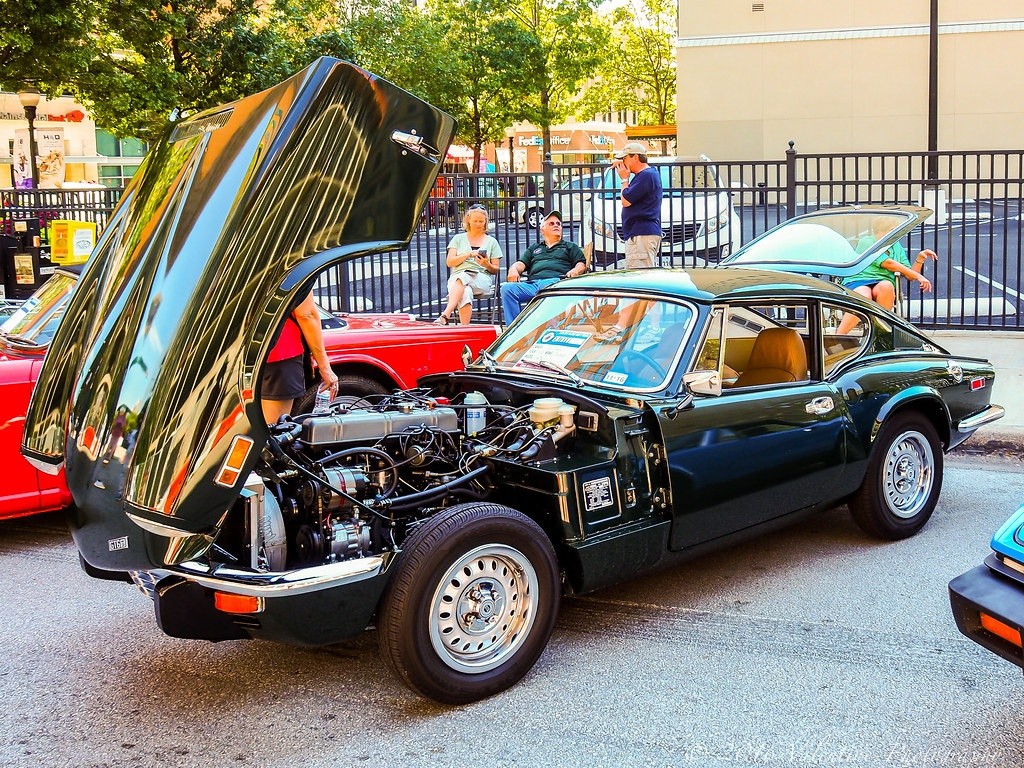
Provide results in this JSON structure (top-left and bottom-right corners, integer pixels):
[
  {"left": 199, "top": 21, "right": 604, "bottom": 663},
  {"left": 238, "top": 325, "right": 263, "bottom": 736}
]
[
  {"left": 834, "top": 216, "right": 938, "bottom": 336},
  {"left": 103, "top": 404, "right": 142, "bottom": 501},
  {"left": 501, "top": 211, "right": 586, "bottom": 328},
  {"left": 433, "top": 208, "right": 504, "bottom": 327},
  {"left": 261, "top": 289, "right": 338, "bottom": 424},
  {"left": 613, "top": 143, "right": 663, "bottom": 270}
]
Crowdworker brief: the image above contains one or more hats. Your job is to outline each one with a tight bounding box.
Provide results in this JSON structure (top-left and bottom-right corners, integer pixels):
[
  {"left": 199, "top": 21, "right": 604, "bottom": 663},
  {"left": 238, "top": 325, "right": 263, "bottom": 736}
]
[
  {"left": 614, "top": 143, "right": 647, "bottom": 160},
  {"left": 540, "top": 210, "right": 562, "bottom": 229}
]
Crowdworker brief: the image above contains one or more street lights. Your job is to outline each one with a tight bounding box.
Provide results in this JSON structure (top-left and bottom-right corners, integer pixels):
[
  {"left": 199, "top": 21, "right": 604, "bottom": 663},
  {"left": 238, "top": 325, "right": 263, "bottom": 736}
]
[{"left": 15, "top": 85, "right": 41, "bottom": 189}]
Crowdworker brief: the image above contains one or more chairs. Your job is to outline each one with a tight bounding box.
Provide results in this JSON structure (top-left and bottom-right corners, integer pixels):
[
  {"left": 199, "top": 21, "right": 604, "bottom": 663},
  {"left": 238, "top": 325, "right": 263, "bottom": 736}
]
[
  {"left": 732, "top": 329, "right": 806, "bottom": 390},
  {"left": 830, "top": 272, "right": 905, "bottom": 331},
  {"left": 448, "top": 268, "right": 501, "bottom": 325},
  {"left": 520, "top": 243, "right": 594, "bottom": 277},
  {"left": 642, "top": 322, "right": 687, "bottom": 382}
]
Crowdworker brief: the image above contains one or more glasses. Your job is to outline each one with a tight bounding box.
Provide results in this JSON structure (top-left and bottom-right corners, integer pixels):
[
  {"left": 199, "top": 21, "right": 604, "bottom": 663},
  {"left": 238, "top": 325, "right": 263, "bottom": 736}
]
[
  {"left": 543, "top": 222, "right": 562, "bottom": 227},
  {"left": 469, "top": 204, "right": 486, "bottom": 210}
]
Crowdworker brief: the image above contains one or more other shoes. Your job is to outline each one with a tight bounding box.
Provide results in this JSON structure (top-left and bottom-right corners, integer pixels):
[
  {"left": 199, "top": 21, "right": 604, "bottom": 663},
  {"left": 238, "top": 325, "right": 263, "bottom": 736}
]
[{"left": 433, "top": 314, "right": 449, "bottom": 326}]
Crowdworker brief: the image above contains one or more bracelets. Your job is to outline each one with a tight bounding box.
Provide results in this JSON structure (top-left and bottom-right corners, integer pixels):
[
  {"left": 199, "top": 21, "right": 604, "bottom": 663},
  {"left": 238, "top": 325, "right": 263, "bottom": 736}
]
[
  {"left": 621, "top": 179, "right": 628, "bottom": 184},
  {"left": 915, "top": 259, "right": 925, "bottom": 264}
]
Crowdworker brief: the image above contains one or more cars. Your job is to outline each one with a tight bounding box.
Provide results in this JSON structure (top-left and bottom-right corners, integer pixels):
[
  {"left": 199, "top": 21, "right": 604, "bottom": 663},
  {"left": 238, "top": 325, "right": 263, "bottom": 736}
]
[
  {"left": 19, "top": 53, "right": 1006, "bottom": 706},
  {"left": 575, "top": 154, "right": 742, "bottom": 271},
  {"left": 511, "top": 172, "right": 602, "bottom": 228},
  {"left": 948, "top": 500, "right": 1023, "bottom": 670},
  {"left": 0, "top": 261, "right": 503, "bottom": 521}
]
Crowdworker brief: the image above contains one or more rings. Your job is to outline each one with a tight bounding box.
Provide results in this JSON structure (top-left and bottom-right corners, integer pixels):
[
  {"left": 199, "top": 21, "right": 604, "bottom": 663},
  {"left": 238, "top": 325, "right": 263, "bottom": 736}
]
[
  {"left": 617, "top": 168, "right": 620, "bottom": 170},
  {"left": 332, "top": 386, "right": 336, "bottom": 390}
]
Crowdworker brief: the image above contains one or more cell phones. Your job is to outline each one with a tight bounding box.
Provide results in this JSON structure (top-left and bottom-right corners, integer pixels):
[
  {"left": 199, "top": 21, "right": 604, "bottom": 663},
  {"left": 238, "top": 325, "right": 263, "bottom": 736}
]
[{"left": 478, "top": 250, "right": 487, "bottom": 259}]
